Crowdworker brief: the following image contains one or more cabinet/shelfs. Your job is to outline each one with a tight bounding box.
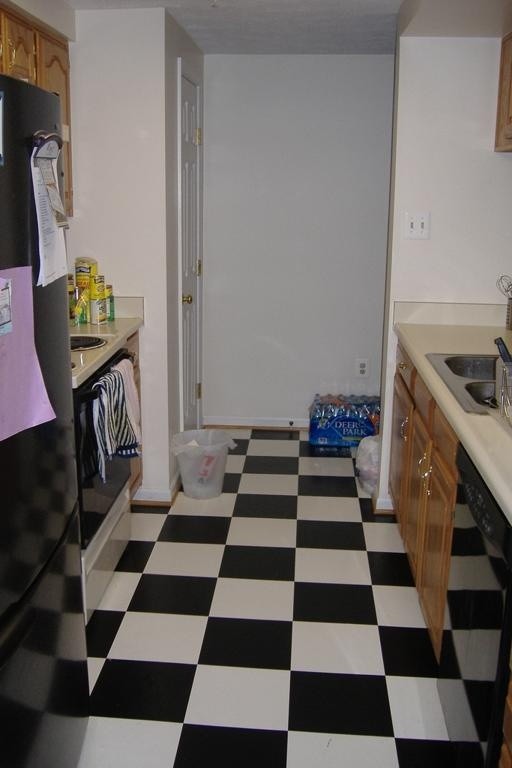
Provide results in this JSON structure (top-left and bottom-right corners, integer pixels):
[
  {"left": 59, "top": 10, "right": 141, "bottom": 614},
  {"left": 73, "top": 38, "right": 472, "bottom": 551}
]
[
  {"left": 0, "top": 1, "right": 73, "bottom": 217},
  {"left": 126, "top": 332, "right": 144, "bottom": 502},
  {"left": 493, "top": 32, "right": 512, "bottom": 152},
  {"left": 417, "top": 403, "right": 467, "bottom": 663},
  {"left": 391, "top": 343, "right": 434, "bottom": 565}
]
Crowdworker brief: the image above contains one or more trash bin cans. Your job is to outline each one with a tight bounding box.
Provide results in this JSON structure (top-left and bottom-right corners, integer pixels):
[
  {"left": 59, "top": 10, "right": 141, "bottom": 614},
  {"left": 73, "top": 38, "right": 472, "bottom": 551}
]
[{"left": 174, "top": 429, "right": 231, "bottom": 499}]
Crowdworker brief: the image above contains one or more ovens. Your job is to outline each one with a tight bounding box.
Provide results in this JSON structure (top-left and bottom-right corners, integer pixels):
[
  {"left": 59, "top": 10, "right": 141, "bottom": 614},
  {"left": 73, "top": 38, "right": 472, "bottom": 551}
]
[{"left": 73, "top": 349, "right": 142, "bottom": 626}]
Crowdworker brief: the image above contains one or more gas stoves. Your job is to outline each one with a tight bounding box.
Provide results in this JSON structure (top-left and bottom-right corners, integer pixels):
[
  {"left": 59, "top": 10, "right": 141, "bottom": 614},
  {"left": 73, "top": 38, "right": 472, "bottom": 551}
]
[{"left": 70, "top": 333, "right": 127, "bottom": 389}]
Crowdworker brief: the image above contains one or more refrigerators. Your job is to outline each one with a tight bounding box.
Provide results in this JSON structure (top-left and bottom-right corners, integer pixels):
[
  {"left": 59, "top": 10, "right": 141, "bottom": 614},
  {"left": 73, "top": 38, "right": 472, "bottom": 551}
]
[{"left": 0, "top": 75, "right": 88, "bottom": 768}]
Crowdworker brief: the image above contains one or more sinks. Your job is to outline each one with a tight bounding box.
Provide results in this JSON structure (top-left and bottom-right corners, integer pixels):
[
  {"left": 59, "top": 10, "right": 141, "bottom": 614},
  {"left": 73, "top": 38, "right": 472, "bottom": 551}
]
[
  {"left": 448, "top": 381, "right": 498, "bottom": 415},
  {"left": 423, "top": 354, "right": 512, "bottom": 379}
]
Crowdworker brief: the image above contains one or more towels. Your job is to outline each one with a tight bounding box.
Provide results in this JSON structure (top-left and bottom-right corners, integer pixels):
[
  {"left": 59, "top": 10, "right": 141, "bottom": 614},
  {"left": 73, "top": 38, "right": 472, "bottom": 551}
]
[
  {"left": 110, "top": 358, "right": 143, "bottom": 456},
  {"left": 80, "top": 402, "right": 97, "bottom": 488},
  {"left": 92, "top": 372, "right": 140, "bottom": 484}
]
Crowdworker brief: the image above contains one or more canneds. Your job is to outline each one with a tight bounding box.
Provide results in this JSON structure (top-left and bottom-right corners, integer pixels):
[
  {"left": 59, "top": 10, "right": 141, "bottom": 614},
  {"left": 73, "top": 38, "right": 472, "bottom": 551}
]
[{"left": 75, "top": 257, "right": 115, "bottom": 327}]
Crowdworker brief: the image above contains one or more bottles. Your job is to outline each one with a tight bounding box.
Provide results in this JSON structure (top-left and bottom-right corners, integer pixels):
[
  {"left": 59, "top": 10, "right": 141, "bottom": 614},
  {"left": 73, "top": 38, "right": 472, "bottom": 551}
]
[
  {"left": 68, "top": 257, "right": 115, "bottom": 325},
  {"left": 309, "top": 393, "right": 382, "bottom": 453}
]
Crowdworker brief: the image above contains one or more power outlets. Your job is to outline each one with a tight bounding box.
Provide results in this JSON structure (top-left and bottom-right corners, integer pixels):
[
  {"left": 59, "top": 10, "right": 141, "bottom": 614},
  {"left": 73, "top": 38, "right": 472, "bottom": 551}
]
[{"left": 405, "top": 213, "right": 430, "bottom": 239}]
